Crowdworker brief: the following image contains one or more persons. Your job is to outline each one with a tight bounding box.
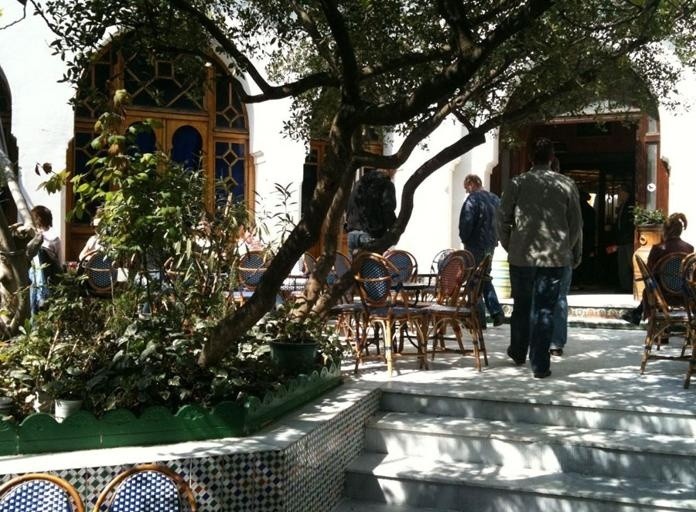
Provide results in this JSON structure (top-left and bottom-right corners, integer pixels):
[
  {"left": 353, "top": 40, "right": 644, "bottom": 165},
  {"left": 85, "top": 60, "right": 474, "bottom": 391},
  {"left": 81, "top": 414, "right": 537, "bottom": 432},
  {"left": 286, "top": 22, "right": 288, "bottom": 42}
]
[
  {"left": 497, "top": 138, "right": 584, "bottom": 380},
  {"left": 343, "top": 162, "right": 396, "bottom": 256},
  {"left": 459, "top": 173, "right": 505, "bottom": 328},
  {"left": 79, "top": 218, "right": 121, "bottom": 259},
  {"left": 27, "top": 205, "right": 63, "bottom": 320},
  {"left": 570, "top": 184, "right": 633, "bottom": 293},
  {"left": 618, "top": 210, "right": 696, "bottom": 327},
  {"left": 546, "top": 153, "right": 573, "bottom": 356}
]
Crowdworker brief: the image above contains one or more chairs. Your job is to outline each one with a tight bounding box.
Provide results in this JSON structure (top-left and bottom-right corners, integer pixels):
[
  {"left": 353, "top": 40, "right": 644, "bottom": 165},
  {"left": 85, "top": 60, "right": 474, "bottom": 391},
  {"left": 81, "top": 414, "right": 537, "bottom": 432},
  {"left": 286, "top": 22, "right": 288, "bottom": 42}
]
[
  {"left": 390, "top": 248, "right": 478, "bottom": 355},
  {"left": 0, "top": 473, "right": 85, "bottom": 512},
  {"left": 350, "top": 250, "right": 430, "bottom": 373},
  {"left": 382, "top": 250, "right": 421, "bottom": 301},
  {"left": 91, "top": 463, "right": 198, "bottom": 512},
  {"left": 301, "top": 250, "right": 368, "bottom": 357},
  {"left": 29, "top": 246, "right": 287, "bottom": 315},
  {"left": 636, "top": 249, "right": 696, "bottom": 391},
  {"left": 416, "top": 250, "right": 494, "bottom": 374}
]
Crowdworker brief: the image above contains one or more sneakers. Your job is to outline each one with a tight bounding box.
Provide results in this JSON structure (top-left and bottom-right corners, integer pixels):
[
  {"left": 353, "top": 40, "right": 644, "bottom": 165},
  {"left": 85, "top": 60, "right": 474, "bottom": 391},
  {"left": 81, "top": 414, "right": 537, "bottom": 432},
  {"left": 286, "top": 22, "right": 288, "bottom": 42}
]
[
  {"left": 494, "top": 314, "right": 505, "bottom": 325},
  {"left": 507, "top": 346, "right": 522, "bottom": 365},
  {"left": 535, "top": 370, "right": 551, "bottom": 378},
  {"left": 623, "top": 311, "right": 641, "bottom": 325}
]
